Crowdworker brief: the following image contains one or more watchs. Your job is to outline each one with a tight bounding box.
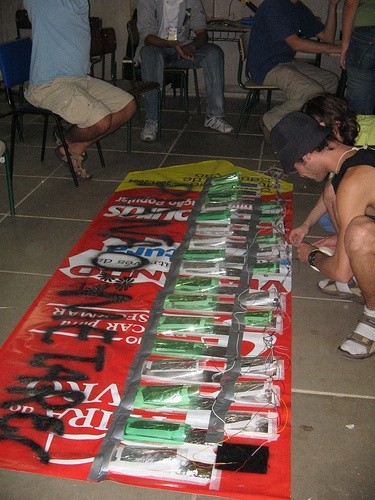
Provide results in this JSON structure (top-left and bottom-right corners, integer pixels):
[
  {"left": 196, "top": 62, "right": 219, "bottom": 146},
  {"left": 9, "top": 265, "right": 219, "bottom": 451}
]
[{"left": 308, "top": 251, "right": 324, "bottom": 266}]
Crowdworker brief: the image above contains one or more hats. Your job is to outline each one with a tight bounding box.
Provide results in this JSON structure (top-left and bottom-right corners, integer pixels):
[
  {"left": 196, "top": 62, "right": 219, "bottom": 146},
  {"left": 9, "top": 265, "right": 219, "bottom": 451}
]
[{"left": 268, "top": 111, "right": 332, "bottom": 174}]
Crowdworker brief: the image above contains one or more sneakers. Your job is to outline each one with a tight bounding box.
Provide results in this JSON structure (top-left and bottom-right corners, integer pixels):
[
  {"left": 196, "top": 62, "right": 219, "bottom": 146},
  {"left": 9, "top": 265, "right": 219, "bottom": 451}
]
[
  {"left": 204, "top": 114, "right": 234, "bottom": 133},
  {"left": 139, "top": 119, "right": 158, "bottom": 142}
]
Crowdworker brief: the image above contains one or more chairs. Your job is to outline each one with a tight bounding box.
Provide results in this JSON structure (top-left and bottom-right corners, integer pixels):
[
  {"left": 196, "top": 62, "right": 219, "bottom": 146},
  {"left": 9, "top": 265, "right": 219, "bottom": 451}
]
[
  {"left": 126, "top": 20, "right": 201, "bottom": 129},
  {"left": 0, "top": 10, "right": 161, "bottom": 216},
  {"left": 235, "top": 33, "right": 283, "bottom": 139}
]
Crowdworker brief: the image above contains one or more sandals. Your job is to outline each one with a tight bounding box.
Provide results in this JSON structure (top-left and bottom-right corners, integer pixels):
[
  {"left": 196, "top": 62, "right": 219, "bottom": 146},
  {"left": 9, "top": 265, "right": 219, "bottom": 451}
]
[
  {"left": 54, "top": 143, "right": 92, "bottom": 182},
  {"left": 336, "top": 312, "right": 375, "bottom": 361},
  {"left": 53, "top": 127, "right": 88, "bottom": 164},
  {"left": 318, "top": 274, "right": 366, "bottom": 305}
]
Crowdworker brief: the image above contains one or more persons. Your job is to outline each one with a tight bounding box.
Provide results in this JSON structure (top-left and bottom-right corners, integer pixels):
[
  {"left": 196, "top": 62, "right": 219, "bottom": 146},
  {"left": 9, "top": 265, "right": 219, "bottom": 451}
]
[
  {"left": 289, "top": 95, "right": 375, "bottom": 253},
  {"left": 340, "top": 0, "right": 375, "bottom": 113},
  {"left": 22, "top": 0, "right": 136, "bottom": 180},
  {"left": 269, "top": 112, "right": 375, "bottom": 361},
  {"left": 133, "top": 0, "right": 236, "bottom": 143},
  {"left": 248, "top": 0, "right": 341, "bottom": 144}
]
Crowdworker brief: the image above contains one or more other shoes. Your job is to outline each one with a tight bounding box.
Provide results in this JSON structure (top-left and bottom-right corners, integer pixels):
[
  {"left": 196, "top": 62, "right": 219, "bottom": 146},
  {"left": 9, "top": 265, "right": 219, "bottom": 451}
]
[{"left": 258, "top": 115, "right": 270, "bottom": 144}]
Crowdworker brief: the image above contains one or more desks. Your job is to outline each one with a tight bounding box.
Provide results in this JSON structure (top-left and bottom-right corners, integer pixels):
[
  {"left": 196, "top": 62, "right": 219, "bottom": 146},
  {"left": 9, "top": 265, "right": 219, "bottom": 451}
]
[{"left": 207, "top": 26, "right": 322, "bottom": 65}]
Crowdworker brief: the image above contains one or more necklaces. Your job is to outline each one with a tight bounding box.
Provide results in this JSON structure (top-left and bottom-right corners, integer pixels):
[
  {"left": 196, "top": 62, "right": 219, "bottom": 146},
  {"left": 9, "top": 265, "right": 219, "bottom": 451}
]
[{"left": 336, "top": 145, "right": 375, "bottom": 173}]
